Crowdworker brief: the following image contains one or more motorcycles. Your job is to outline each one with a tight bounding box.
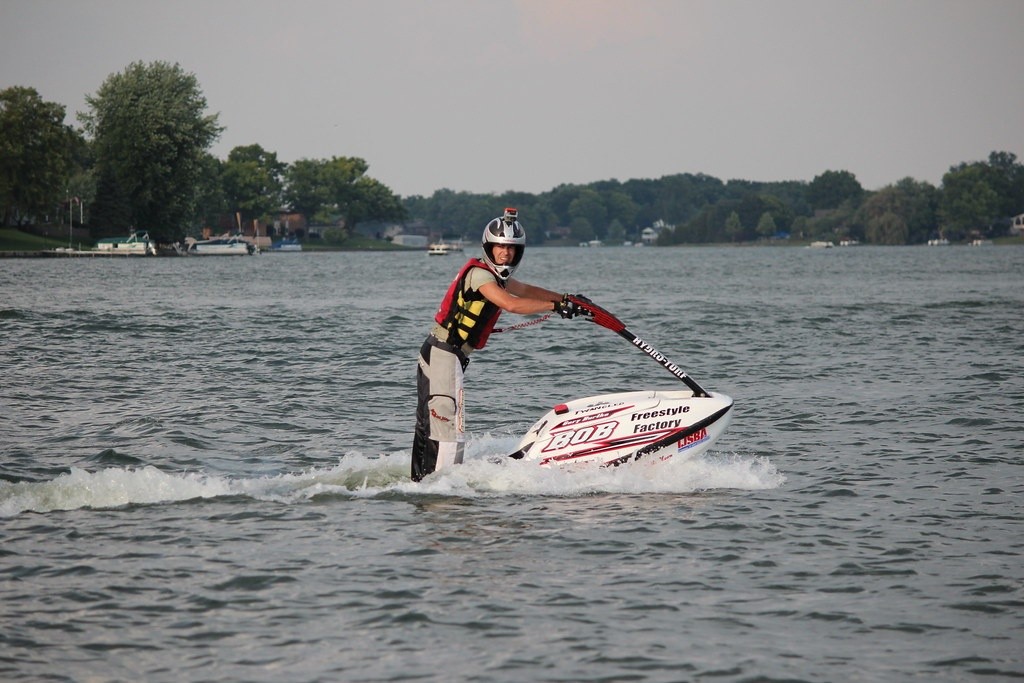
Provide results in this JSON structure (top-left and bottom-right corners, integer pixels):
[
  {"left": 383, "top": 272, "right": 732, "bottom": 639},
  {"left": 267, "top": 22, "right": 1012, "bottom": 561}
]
[{"left": 406, "top": 295, "right": 736, "bottom": 491}]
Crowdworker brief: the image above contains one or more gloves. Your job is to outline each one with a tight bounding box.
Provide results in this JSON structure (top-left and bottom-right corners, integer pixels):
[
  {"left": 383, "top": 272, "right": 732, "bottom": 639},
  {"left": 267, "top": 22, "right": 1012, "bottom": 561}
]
[{"left": 551, "top": 292, "right": 592, "bottom": 320}]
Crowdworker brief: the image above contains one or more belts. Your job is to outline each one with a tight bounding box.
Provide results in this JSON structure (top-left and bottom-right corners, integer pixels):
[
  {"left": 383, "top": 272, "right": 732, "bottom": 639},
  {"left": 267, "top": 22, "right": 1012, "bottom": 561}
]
[{"left": 426, "top": 335, "right": 470, "bottom": 373}]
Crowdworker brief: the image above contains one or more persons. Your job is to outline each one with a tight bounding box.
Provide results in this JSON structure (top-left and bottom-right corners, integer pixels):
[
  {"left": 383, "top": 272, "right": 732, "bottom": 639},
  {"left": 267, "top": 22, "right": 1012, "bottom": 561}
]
[{"left": 409, "top": 208, "right": 590, "bottom": 484}]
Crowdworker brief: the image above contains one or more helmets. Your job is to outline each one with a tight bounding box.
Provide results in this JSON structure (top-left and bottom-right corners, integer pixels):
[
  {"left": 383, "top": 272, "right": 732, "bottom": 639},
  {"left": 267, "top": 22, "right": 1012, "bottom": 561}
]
[{"left": 481, "top": 208, "right": 526, "bottom": 281}]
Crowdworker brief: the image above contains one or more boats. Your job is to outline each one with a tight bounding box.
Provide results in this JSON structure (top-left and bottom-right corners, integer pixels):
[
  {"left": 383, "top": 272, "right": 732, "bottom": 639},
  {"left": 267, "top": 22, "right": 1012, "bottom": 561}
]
[{"left": 427, "top": 242, "right": 449, "bottom": 254}]
